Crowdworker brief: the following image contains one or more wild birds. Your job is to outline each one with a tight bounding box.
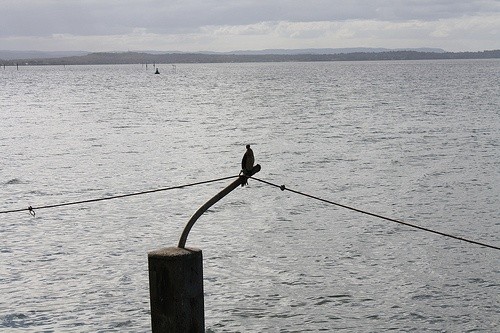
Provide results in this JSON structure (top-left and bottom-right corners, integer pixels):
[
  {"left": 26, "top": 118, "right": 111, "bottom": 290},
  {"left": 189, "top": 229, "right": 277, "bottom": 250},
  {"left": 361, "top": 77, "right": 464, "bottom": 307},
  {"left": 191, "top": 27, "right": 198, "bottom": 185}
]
[{"left": 239, "top": 145, "right": 254, "bottom": 187}]
